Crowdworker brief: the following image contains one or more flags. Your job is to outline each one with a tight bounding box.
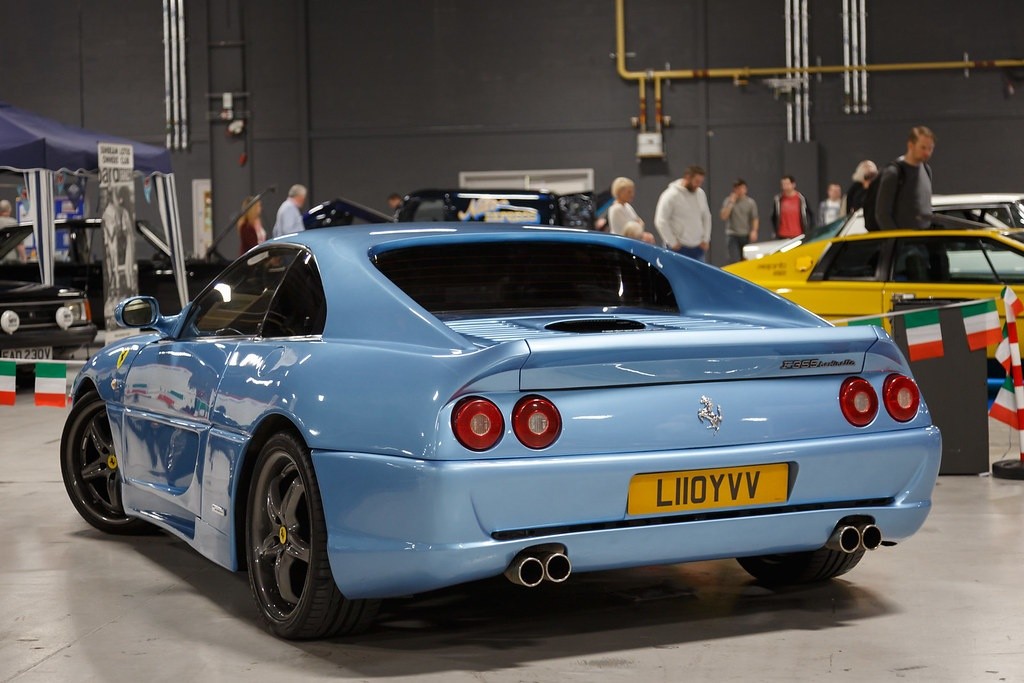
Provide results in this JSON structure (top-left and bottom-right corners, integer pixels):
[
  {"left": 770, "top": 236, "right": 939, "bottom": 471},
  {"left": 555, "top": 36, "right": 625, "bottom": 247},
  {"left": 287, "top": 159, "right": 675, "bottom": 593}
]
[{"left": 847, "top": 285, "right": 1024, "bottom": 431}]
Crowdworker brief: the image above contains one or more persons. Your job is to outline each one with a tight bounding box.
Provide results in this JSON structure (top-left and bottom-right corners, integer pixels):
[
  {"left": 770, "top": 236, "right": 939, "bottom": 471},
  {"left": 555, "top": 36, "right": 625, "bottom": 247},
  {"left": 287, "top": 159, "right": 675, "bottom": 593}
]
[
  {"left": 877, "top": 125, "right": 936, "bottom": 231},
  {"left": 102, "top": 185, "right": 137, "bottom": 303},
  {"left": 720, "top": 178, "right": 760, "bottom": 264},
  {"left": 594, "top": 165, "right": 715, "bottom": 263},
  {"left": 815, "top": 159, "right": 879, "bottom": 229},
  {"left": 237, "top": 184, "right": 308, "bottom": 296},
  {"left": 768, "top": 176, "right": 812, "bottom": 246},
  {"left": 0, "top": 200, "right": 28, "bottom": 263},
  {"left": 388, "top": 193, "right": 403, "bottom": 210}
]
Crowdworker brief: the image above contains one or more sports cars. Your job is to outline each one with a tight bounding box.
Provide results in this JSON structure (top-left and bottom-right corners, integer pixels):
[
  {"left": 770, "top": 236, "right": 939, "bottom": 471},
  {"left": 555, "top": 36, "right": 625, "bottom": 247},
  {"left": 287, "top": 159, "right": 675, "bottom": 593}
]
[{"left": 57, "top": 213, "right": 942, "bottom": 643}]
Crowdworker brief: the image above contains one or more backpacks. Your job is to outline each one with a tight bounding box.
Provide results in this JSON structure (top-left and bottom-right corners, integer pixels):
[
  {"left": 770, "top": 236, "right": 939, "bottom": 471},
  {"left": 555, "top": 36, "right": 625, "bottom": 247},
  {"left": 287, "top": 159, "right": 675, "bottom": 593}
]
[{"left": 863, "top": 163, "right": 904, "bottom": 231}]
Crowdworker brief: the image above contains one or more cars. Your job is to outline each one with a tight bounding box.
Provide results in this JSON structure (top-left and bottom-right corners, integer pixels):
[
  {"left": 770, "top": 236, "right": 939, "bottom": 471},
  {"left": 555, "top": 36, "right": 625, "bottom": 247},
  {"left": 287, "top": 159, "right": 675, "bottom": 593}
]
[
  {"left": 0, "top": 216, "right": 101, "bottom": 357},
  {"left": 706, "top": 215, "right": 1023, "bottom": 387},
  {"left": 739, "top": 193, "right": 1023, "bottom": 283}
]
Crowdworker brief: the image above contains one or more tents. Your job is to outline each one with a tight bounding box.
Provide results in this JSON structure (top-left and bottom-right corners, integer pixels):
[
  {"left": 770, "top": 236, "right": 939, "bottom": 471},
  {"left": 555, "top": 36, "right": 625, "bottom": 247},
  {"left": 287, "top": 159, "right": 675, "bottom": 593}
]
[{"left": 0, "top": 105, "right": 189, "bottom": 310}]
